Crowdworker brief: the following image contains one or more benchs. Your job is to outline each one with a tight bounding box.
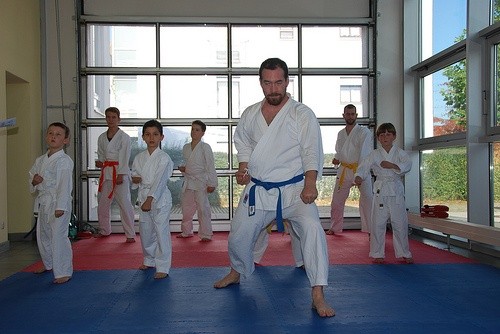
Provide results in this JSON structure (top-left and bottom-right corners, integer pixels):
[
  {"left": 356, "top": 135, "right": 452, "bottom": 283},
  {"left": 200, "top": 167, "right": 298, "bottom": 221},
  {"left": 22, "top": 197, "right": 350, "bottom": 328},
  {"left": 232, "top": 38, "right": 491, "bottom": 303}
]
[{"left": 408, "top": 215, "right": 500, "bottom": 249}]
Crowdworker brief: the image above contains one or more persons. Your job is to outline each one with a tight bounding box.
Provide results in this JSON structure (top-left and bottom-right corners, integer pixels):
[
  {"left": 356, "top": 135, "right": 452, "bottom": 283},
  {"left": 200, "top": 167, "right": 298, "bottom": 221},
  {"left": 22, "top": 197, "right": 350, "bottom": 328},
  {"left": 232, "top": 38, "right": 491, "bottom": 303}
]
[
  {"left": 213, "top": 57, "right": 338, "bottom": 318},
  {"left": 26, "top": 123, "right": 75, "bottom": 282},
  {"left": 130, "top": 120, "right": 173, "bottom": 278},
  {"left": 178, "top": 120, "right": 219, "bottom": 243},
  {"left": 325, "top": 104, "right": 373, "bottom": 235},
  {"left": 351, "top": 122, "right": 413, "bottom": 264},
  {"left": 94, "top": 107, "right": 135, "bottom": 244}
]
